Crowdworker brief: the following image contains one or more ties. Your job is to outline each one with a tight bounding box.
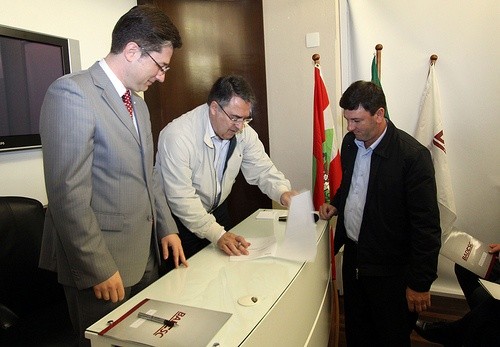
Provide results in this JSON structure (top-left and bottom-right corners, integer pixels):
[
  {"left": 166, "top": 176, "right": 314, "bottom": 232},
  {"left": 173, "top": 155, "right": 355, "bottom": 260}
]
[{"left": 122, "top": 90, "right": 133, "bottom": 120}]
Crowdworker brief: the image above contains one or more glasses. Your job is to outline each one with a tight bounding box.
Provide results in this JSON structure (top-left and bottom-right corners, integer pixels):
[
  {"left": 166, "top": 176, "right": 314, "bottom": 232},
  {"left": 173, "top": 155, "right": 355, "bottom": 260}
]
[
  {"left": 126, "top": 40, "right": 170, "bottom": 76},
  {"left": 211, "top": 99, "right": 253, "bottom": 125}
]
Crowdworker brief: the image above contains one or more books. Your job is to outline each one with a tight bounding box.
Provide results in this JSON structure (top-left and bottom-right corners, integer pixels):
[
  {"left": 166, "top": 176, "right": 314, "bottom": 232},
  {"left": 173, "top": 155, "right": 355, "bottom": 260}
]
[
  {"left": 99, "top": 298, "right": 233, "bottom": 347},
  {"left": 440, "top": 228, "right": 497, "bottom": 279}
]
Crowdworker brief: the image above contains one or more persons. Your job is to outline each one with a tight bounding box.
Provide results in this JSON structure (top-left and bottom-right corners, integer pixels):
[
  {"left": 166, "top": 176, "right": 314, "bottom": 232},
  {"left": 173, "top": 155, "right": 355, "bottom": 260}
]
[
  {"left": 319, "top": 80, "right": 442, "bottom": 347},
  {"left": 38, "top": 5, "right": 189, "bottom": 347},
  {"left": 155, "top": 73, "right": 302, "bottom": 256},
  {"left": 416, "top": 242, "right": 500, "bottom": 347}
]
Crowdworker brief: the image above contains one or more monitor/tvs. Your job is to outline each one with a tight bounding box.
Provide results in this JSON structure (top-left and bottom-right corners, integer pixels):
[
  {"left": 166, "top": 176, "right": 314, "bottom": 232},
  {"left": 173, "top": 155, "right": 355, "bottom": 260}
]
[{"left": 0, "top": 24, "right": 81, "bottom": 151}]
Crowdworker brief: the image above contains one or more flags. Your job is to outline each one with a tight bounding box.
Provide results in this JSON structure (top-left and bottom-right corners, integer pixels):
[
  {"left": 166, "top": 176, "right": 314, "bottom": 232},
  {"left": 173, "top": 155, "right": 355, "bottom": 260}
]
[
  {"left": 371, "top": 54, "right": 390, "bottom": 120},
  {"left": 312, "top": 63, "right": 345, "bottom": 282},
  {"left": 414, "top": 61, "right": 458, "bottom": 236}
]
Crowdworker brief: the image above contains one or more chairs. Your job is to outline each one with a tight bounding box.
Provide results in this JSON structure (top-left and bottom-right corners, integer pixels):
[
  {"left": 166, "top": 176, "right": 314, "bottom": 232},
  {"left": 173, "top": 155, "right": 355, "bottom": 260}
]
[{"left": 0, "top": 196, "right": 75, "bottom": 347}]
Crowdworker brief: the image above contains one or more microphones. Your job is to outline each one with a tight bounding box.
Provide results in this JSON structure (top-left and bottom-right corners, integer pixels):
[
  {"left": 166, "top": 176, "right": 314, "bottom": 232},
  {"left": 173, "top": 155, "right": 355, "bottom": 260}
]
[{"left": 279, "top": 214, "right": 319, "bottom": 223}]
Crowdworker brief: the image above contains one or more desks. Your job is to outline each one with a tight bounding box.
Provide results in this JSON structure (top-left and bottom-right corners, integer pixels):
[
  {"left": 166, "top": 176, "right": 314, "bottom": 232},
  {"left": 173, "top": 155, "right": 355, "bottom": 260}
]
[{"left": 83, "top": 208, "right": 331, "bottom": 347}]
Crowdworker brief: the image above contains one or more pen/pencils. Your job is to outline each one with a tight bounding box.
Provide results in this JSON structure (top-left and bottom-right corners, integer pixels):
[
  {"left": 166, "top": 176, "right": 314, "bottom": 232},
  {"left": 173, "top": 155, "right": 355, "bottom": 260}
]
[{"left": 137, "top": 311, "right": 180, "bottom": 329}]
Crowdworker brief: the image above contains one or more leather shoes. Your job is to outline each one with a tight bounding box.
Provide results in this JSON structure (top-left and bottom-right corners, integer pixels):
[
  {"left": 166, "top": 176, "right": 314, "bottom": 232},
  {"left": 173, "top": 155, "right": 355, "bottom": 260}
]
[{"left": 413, "top": 319, "right": 445, "bottom": 345}]
